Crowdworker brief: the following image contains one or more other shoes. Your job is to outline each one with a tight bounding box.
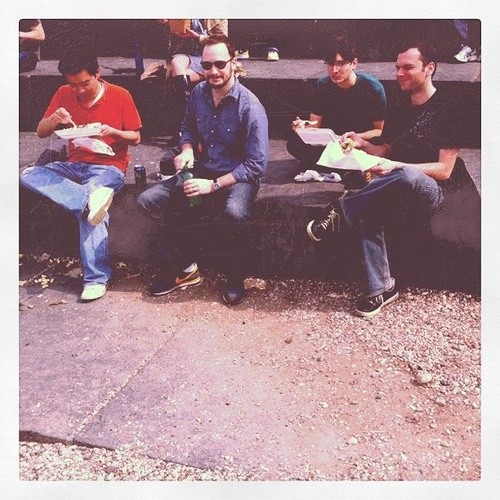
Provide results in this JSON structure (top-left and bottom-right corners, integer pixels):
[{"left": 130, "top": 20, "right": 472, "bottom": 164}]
[
  {"left": 80, "top": 282, "right": 107, "bottom": 301},
  {"left": 86, "top": 185, "right": 115, "bottom": 227}
]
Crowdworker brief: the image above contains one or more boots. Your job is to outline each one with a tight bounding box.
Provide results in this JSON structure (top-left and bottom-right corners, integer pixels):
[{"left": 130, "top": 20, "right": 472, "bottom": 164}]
[{"left": 172, "top": 73, "right": 191, "bottom": 124}]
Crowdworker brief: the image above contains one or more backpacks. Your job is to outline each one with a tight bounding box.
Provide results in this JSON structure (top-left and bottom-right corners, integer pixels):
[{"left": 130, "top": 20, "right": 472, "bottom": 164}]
[{"left": 135, "top": 63, "right": 168, "bottom": 136}]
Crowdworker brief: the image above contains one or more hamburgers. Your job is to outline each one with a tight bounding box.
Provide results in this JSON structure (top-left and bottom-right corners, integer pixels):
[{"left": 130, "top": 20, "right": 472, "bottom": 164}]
[{"left": 341, "top": 138, "right": 354, "bottom": 153}]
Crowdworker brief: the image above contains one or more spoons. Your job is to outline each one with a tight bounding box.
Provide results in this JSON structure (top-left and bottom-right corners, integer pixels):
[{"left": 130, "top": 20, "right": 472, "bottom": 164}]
[{"left": 302, "top": 120, "right": 318, "bottom": 125}]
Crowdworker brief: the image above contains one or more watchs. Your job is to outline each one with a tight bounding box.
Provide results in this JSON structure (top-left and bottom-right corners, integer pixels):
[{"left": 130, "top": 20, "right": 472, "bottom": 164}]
[{"left": 212, "top": 178, "right": 221, "bottom": 191}]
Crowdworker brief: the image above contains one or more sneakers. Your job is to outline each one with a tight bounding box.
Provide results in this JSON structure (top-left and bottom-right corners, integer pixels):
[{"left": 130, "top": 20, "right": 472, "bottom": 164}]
[
  {"left": 220, "top": 264, "right": 248, "bottom": 305},
  {"left": 149, "top": 262, "right": 202, "bottom": 296},
  {"left": 306, "top": 200, "right": 342, "bottom": 243},
  {"left": 452, "top": 45, "right": 478, "bottom": 63},
  {"left": 354, "top": 287, "right": 399, "bottom": 318},
  {"left": 267, "top": 49, "right": 279, "bottom": 61},
  {"left": 235, "top": 48, "right": 250, "bottom": 59}
]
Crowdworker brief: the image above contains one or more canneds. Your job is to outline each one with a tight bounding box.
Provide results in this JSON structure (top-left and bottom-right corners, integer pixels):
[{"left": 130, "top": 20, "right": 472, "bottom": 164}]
[{"left": 134, "top": 165, "right": 146, "bottom": 186}]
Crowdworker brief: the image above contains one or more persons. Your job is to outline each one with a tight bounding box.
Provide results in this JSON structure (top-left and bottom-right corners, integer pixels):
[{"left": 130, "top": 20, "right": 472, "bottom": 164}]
[
  {"left": 287, "top": 33, "right": 385, "bottom": 182},
  {"left": 19, "top": 39, "right": 143, "bottom": 301},
  {"left": 19, "top": 19, "right": 45, "bottom": 74},
  {"left": 305, "top": 39, "right": 467, "bottom": 318},
  {"left": 160, "top": 19, "right": 283, "bottom": 137},
  {"left": 453, "top": 19, "right": 481, "bottom": 64},
  {"left": 139, "top": 35, "right": 269, "bottom": 304}
]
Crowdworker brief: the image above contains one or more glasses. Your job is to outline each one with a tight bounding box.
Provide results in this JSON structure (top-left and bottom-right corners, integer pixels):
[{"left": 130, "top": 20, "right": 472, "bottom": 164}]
[
  {"left": 200, "top": 56, "right": 233, "bottom": 70},
  {"left": 324, "top": 59, "right": 351, "bottom": 69}
]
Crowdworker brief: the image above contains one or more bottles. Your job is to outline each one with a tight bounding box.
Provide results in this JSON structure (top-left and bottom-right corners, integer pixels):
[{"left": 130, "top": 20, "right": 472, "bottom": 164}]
[{"left": 183, "top": 164, "right": 202, "bottom": 207}]
